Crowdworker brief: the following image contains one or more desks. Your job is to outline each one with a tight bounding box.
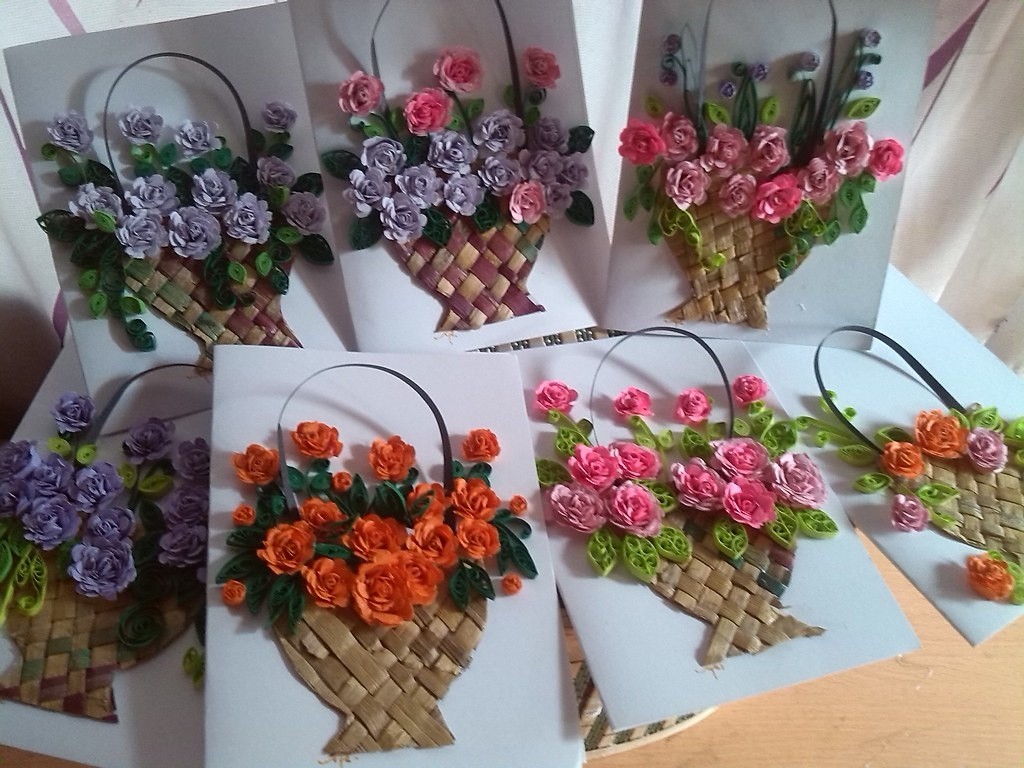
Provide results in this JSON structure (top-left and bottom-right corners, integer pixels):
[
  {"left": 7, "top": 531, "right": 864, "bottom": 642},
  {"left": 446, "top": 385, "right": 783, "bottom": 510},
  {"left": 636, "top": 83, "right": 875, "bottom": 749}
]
[{"left": 0, "top": 525, "right": 1024, "bottom": 768}]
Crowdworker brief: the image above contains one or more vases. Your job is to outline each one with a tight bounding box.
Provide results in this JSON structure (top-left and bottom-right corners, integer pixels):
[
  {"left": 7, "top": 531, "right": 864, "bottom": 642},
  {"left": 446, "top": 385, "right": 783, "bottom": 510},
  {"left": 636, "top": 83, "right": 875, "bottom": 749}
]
[
  {"left": 615, "top": 504, "right": 827, "bottom": 667},
  {"left": 119, "top": 248, "right": 303, "bottom": 375},
  {"left": 395, "top": 213, "right": 549, "bottom": 332},
  {"left": 879, "top": 448, "right": 1024, "bottom": 554},
  {"left": 267, "top": 592, "right": 487, "bottom": 751},
  {"left": 651, "top": 168, "right": 833, "bottom": 329}
]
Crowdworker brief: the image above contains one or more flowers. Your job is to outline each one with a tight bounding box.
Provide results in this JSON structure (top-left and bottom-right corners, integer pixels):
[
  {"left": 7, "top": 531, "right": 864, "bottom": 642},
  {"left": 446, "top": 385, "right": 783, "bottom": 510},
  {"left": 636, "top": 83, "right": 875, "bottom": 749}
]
[
  {"left": 797, "top": 390, "right": 1024, "bottom": 604},
  {"left": 215, "top": 422, "right": 538, "bottom": 633},
  {"left": 0, "top": 392, "right": 210, "bottom": 685},
  {"left": 532, "top": 375, "right": 840, "bottom": 582},
  {"left": 29, "top": 103, "right": 334, "bottom": 349},
  {"left": 617, "top": 28, "right": 904, "bottom": 270},
  {"left": 321, "top": 45, "right": 595, "bottom": 247}
]
